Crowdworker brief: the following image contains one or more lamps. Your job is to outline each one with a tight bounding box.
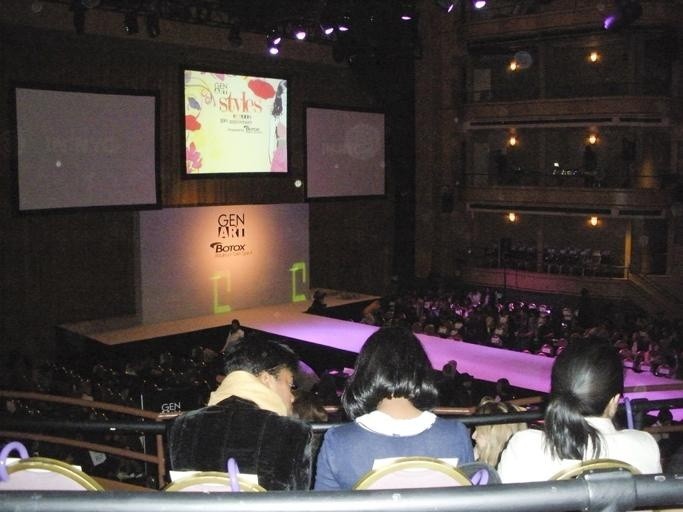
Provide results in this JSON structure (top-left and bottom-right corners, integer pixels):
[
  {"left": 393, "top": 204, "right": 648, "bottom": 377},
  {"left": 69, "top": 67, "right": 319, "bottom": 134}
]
[{"left": 263, "top": 1, "right": 488, "bottom": 55}]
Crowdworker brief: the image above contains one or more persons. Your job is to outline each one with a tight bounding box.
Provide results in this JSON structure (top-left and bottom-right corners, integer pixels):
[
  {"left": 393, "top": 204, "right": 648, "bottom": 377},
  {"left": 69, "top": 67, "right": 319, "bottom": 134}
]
[
  {"left": 490, "top": 300, "right": 683, "bottom": 378},
  {"left": 449, "top": 360, "right": 460, "bottom": 376},
  {"left": 362, "top": 288, "right": 503, "bottom": 345},
  {"left": 478, "top": 378, "right": 513, "bottom": 409},
  {"left": 496, "top": 336, "right": 663, "bottom": 484},
  {"left": 471, "top": 402, "right": 528, "bottom": 469},
  {"left": 165, "top": 334, "right": 314, "bottom": 492},
  {"left": 649, "top": 408, "right": 673, "bottom": 443},
  {"left": 308, "top": 288, "right": 329, "bottom": 315},
  {"left": 313, "top": 326, "right": 474, "bottom": 492},
  {"left": 442, "top": 364, "right": 452, "bottom": 378},
  {"left": 220, "top": 319, "right": 245, "bottom": 353},
  {"left": 480, "top": 241, "right": 610, "bottom": 277},
  {"left": 462, "top": 373, "right": 473, "bottom": 394}
]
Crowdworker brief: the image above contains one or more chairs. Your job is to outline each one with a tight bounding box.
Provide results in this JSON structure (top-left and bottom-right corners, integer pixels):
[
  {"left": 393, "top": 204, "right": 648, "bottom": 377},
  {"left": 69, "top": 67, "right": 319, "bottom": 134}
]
[
  {"left": 352, "top": 458, "right": 471, "bottom": 490},
  {"left": 161, "top": 472, "right": 268, "bottom": 492},
  {"left": 413, "top": 292, "right": 658, "bottom": 368},
  {"left": 548, "top": 459, "right": 644, "bottom": 479},
  {"left": 6, "top": 457, "right": 105, "bottom": 491}
]
